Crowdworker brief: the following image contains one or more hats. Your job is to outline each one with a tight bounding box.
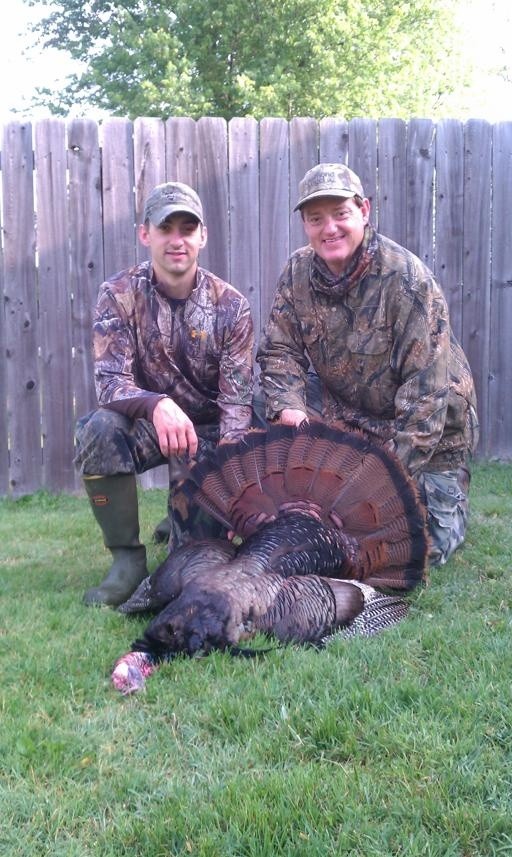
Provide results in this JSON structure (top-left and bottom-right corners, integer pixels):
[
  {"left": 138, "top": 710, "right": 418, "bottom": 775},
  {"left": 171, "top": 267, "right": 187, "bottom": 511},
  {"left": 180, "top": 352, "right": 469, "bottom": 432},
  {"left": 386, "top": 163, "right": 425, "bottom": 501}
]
[
  {"left": 292, "top": 162, "right": 365, "bottom": 213},
  {"left": 143, "top": 183, "right": 204, "bottom": 228}
]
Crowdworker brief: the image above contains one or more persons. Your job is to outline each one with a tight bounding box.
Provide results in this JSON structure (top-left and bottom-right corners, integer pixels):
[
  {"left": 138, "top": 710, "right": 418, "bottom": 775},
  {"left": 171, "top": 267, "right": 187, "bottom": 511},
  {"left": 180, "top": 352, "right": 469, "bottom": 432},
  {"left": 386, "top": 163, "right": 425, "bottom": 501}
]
[
  {"left": 72, "top": 181, "right": 265, "bottom": 609},
  {"left": 255, "top": 163, "right": 480, "bottom": 569}
]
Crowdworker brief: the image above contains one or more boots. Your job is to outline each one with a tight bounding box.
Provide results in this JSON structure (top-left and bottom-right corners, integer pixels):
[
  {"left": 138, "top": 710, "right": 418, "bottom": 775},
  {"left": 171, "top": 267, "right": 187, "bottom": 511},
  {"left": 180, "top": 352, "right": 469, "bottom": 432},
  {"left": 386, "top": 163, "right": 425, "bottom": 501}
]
[{"left": 82, "top": 474, "right": 148, "bottom": 609}]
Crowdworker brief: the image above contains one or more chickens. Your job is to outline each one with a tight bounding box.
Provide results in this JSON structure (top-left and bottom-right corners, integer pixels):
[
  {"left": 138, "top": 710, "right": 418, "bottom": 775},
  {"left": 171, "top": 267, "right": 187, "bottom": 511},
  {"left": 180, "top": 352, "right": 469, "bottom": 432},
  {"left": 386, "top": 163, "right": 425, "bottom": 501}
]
[{"left": 110, "top": 416, "right": 434, "bottom": 697}]
